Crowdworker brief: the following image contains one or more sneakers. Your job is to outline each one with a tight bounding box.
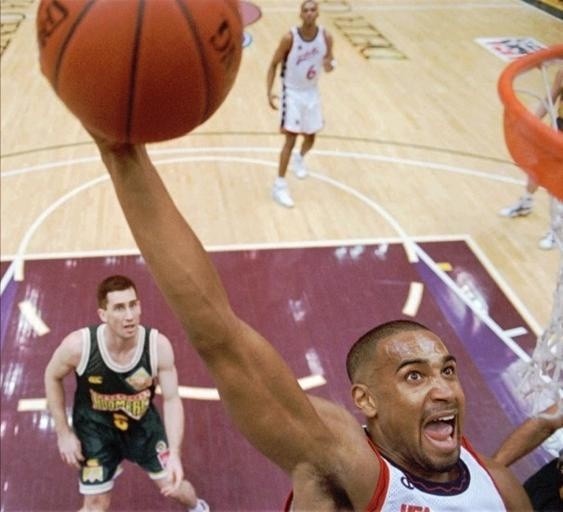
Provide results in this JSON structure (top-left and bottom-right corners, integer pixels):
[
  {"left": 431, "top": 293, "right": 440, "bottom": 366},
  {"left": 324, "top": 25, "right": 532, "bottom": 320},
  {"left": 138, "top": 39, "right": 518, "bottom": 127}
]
[
  {"left": 187, "top": 500, "right": 210, "bottom": 512},
  {"left": 294, "top": 152, "right": 307, "bottom": 178},
  {"left": 273, "top": 186, "right": 293, "bottom": 207},
  {"left": 538, "top": 233, "right": 558, "bottom": 250},
  {"left": 499, "top": 202, "right": 531, "bottom": 218}
]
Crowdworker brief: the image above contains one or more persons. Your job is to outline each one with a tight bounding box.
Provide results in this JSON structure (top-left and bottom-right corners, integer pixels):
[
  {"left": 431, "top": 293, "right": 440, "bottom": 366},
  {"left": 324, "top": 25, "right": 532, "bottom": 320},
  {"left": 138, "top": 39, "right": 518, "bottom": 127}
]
[
  {"left": 266, "top": 0, "right": 336, "bottom": 210},
  {"left": 83, "top": 120, "right": 534, "bottom": 511},
  {"left": 492, "top": 398, "right": 562, "bottom": 510},
  {"left": 42, "top": 274, "right": 208, "bottom": 510},
  {"left": 499, "top": 67, "right": 562, "bottom": 249}
]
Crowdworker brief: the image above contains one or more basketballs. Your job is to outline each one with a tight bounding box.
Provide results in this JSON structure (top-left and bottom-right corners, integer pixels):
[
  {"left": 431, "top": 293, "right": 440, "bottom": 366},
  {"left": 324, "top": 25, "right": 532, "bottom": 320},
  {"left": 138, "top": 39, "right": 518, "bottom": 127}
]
[{"left": 37, "top": 1, "right": 241, "bottom": 143}]
